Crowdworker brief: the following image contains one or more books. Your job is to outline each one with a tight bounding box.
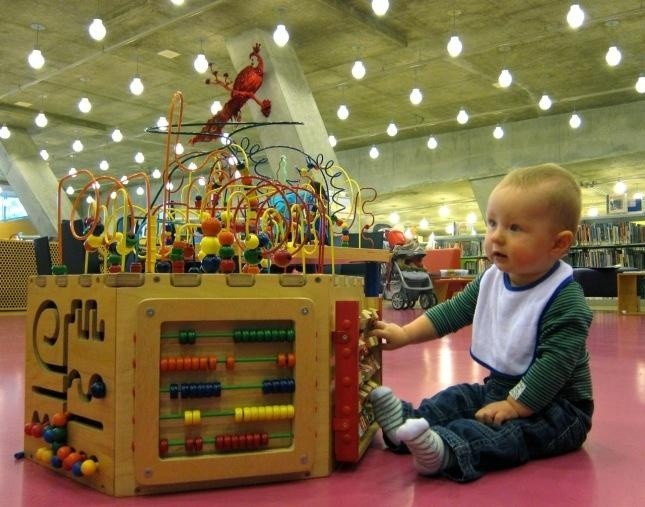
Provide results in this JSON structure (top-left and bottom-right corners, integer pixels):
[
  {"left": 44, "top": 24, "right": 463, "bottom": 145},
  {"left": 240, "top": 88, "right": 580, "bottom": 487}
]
[
  {"left": 433, "top": 239, "right": 493, "bottom": 275},
  {"left": 561, "top": 220, "right": 645, "bottom": 271}
]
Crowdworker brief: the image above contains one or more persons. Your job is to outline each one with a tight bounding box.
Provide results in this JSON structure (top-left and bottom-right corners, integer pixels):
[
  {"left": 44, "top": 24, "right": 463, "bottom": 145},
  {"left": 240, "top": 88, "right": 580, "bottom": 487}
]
[{"left": 369, "top": 163, "right": 594, "bottom": 486}]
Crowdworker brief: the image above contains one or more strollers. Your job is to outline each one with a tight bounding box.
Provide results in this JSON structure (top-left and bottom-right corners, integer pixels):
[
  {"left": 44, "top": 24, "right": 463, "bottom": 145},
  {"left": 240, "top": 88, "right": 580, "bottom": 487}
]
[{"left": 382, "top": 249, "right": 437, "bottom": 310}]
